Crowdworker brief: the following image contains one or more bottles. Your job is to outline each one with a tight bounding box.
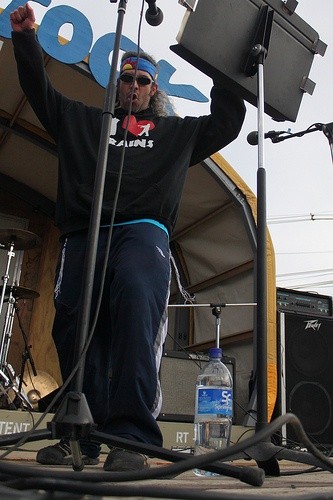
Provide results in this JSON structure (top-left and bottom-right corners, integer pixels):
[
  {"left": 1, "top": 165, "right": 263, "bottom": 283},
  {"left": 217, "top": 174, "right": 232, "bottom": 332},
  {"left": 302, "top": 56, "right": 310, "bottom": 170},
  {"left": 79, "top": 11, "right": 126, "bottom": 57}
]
[{"left": 194, "top": 348, "right": 233, "bottom": 478}]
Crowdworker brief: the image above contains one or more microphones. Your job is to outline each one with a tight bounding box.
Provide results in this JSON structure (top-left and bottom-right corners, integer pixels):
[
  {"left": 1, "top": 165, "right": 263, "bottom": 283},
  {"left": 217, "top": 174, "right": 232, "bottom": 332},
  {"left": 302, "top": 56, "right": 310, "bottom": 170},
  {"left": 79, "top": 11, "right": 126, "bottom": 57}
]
[
  {"left": 145, "top": 0, "right": 163, "bottom": 27},
  {"left": 247, "top": 130, "right": 286, "bottom": 146}
]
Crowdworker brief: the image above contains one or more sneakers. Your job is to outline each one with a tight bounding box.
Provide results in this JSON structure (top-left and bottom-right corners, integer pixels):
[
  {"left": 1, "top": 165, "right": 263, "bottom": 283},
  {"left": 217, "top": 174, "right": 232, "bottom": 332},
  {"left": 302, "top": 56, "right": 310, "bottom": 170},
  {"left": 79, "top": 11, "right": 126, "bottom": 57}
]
[
  {"left": 36, "top": 440, "right": 99, "bottom": 464},
  {"left": 103, "top": 448, "right": 149, "bottom": 471}
]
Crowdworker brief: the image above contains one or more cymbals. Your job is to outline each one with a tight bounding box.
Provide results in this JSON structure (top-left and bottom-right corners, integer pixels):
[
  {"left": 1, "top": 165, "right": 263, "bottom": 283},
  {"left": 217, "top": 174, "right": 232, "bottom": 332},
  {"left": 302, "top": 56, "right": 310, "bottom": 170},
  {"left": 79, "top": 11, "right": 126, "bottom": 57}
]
[
  {"left": 8, "top": 369, "right": 59, "bottom": 409},
  {"left": 0, "top": 229, "right": 42, "bottom": 251},
  {"left": 0, "top": 285, "right": 40, "bottom": 299}
]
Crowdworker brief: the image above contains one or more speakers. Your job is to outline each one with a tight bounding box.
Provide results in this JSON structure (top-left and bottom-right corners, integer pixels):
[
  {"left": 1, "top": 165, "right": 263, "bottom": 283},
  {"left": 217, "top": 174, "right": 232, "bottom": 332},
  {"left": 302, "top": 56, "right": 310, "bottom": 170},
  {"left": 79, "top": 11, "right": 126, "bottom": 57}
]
[
  {"left": 155, "top": 350, "right": 237, "bottom": 426},
  {"left": 271, "top": 288, "right": 333, "bottom": 453}
]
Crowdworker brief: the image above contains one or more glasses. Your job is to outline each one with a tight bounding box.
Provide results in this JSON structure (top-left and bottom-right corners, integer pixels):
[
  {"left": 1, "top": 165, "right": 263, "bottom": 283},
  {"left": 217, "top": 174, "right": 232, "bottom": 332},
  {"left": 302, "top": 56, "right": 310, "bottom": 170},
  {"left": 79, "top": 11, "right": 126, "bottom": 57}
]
[{"left": 119, "top": 74, "right": 154, "bottom": 85}]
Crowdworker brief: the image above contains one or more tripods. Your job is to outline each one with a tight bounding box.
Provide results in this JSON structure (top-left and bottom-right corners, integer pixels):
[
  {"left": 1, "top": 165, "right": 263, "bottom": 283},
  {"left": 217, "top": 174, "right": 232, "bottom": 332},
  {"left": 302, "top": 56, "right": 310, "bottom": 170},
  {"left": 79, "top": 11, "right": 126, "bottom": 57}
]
[{"left": 0, "top": 0, "right": 265, "bottom": 488}]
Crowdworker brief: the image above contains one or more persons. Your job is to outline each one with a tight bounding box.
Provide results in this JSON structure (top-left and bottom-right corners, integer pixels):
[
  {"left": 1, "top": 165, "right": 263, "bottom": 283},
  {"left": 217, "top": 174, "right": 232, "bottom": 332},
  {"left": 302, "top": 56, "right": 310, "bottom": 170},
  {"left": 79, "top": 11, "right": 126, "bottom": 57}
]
[{"left": 9, "top": 2, "right": 247, "bottom": 472}]
